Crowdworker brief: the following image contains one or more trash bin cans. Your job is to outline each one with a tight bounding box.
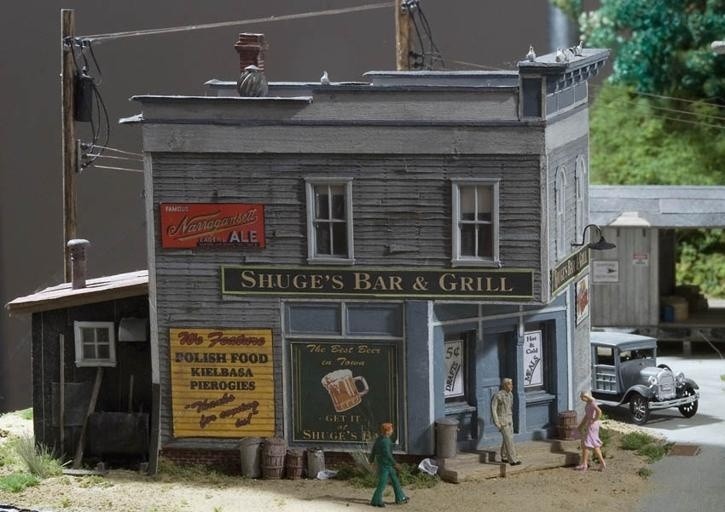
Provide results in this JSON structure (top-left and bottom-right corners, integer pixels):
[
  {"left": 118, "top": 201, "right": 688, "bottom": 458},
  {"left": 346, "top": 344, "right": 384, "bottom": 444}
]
[
  {"left": 307, "top": 446, "right": 325, "bottom": 478},
  {"left": 237, "top": 436, "right": 262, "bottom": 479},
  {"left": 434, "top": 417, "right": 460, "bottom": 457}
]
